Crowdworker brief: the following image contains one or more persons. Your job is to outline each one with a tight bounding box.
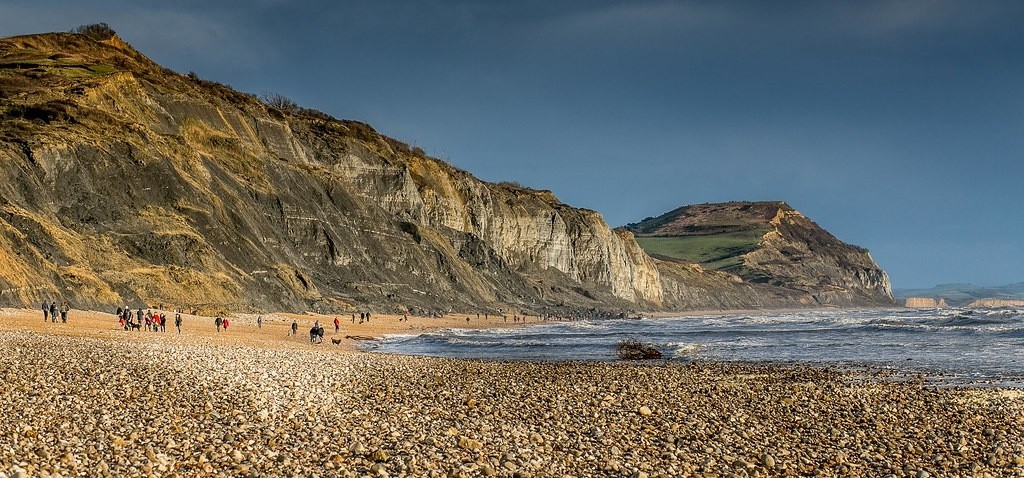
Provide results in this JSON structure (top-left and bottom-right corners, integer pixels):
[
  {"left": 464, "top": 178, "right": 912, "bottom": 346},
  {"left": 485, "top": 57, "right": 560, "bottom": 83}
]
[
  {"left": 485, "top": 312, "right": 488, "bottom": 319},
  {"left": 117, "top": 302, "right": 166, "bottom": 332},
  {"left": 539, "top": 310, "right": 575, "bottom": 322},
  {"left": 334, "top": 317, "right": 340, "bottom": 333},
  {"left": 466, "top": 317, "right": 471, "bottom": 324},
  {"left": 258, "top": 316, "right": 262, "bottom": 329},
  {"left": 42, "top": 300, "right": 70, "bottom": 324},
  {"left": 173, "top": 306, "right": 183, "bottom": 334},
  {"left": 477, "top": 312, "right": 480, "bottom": 318},
  {"left": 215, "top": 315, "right": 229, "bottom": 332},
  {"left": 504, "top": 316, "right": 525, "bottom": 323},
  {"left": 404, "top": 312, "right": 407, "bottom": 322},
  {"left": 291, "top": 320, "right": 297, "bottom": 334},
  {"left": 309, "top": 320, "right": 324, "bottom": 343},
  {"left": 352, "top": 311, "right": 371, "bottom": 323}
]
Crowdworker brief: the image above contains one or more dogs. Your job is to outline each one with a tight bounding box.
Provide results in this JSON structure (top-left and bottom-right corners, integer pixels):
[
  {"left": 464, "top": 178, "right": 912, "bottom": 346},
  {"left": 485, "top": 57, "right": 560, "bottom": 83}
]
[{"left": 331, "top": 337, "right": 341, "bottom": 345}]
